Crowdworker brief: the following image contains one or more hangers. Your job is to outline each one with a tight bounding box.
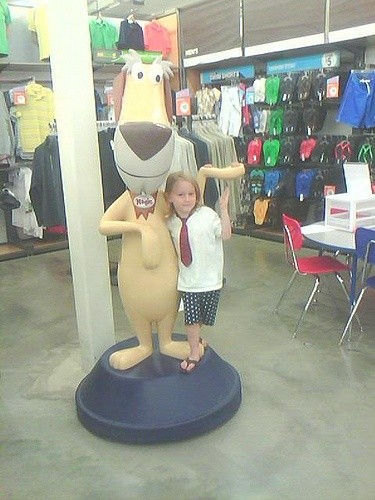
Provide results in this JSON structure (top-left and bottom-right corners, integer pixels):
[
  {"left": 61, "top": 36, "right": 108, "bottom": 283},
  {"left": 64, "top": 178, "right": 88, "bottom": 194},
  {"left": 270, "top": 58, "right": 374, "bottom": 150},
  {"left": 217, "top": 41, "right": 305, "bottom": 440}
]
[
  {"left": 25, "top": 75, "right": 45, "bottom": 96},
  {"left": 126, "top": 9, "right": 136, "bottom": 24},
  {"left": 48, "top": 121, "right": 58, "bottom": 151},
  {"left": 95, "top": 10, "right": 104, "bottom": 23},
  {"left": 97, "top": 120, "right": 118, "bottom": 133},
  {"left": 350, "top": 62, "right": 374, "bottom": 75},
  {"left": 171, "top": 113, "right": 223, "bottom": 136},
  {"left": 151, "top": 14, "right": 160, "bottom": 27}
]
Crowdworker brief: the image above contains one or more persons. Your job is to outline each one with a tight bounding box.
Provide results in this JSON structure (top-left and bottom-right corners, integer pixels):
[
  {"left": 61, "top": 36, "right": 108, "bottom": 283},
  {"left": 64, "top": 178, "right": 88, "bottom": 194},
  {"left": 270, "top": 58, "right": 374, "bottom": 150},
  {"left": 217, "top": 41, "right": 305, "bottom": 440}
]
[{"left": 163, "top": 171, "right": 231, "bottom": 375}]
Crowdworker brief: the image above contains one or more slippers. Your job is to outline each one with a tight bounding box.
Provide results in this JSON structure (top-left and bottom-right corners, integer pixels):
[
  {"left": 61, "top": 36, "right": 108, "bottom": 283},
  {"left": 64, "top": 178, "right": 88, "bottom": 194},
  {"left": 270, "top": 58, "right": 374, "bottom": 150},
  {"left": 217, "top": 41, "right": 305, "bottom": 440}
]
[
  {"left": 179, "top": 337, "right": 207, "bottom": 373},
  {"left": 245, "top": 71, "right": 375, "bottom": 225}
]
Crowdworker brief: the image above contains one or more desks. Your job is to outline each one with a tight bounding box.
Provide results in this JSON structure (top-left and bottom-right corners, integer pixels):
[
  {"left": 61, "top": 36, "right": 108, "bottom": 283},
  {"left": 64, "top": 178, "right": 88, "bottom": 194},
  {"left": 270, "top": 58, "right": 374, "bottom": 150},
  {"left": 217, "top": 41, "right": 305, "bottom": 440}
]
[{"left": 300, "top": 220, "right": 373, "bottom": 303}]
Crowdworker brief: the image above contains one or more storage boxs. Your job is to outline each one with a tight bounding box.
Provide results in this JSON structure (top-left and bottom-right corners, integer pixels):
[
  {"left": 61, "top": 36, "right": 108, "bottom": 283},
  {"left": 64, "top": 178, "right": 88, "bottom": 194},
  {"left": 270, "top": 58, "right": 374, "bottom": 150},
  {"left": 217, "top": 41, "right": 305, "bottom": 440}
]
[{"left": 325, "top": 192, "right": 375, "bottom": 233}]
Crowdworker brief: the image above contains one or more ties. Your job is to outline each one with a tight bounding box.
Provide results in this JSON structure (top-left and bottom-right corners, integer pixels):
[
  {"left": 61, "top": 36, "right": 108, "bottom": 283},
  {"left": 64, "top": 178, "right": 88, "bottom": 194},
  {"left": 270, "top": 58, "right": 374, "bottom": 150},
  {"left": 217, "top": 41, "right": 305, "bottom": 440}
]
[{"left": 180, "top": 219, "right": 192, "bottom": 267}]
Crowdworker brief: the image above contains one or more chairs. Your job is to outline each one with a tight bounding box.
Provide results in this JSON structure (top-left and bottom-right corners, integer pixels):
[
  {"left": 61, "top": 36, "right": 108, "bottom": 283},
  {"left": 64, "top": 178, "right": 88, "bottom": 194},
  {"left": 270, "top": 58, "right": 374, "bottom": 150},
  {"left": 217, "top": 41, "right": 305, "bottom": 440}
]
[
  {"left": 338, "top": 227, "right": 375, "bottom": 346},
  {"left": 273, "top": 214, "right": 365, "bottom": 338}
]
